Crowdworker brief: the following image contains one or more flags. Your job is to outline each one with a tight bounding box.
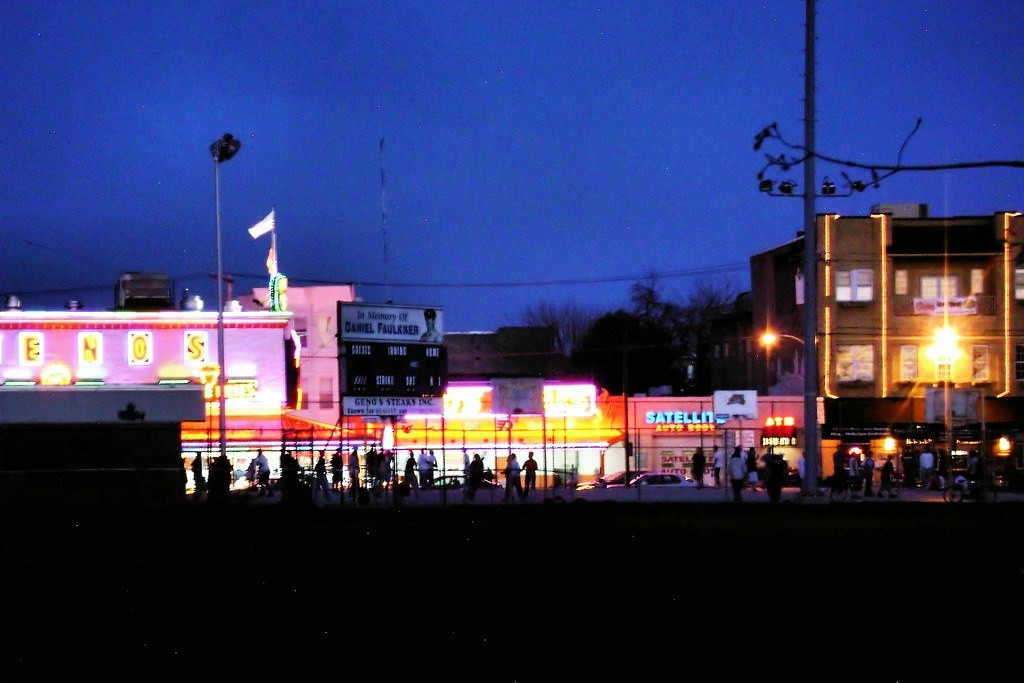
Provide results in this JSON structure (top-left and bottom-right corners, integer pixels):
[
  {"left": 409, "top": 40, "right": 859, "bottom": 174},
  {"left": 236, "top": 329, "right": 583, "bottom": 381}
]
[{"left": 247, "top": 209, "right": 275, "bottom": 240}]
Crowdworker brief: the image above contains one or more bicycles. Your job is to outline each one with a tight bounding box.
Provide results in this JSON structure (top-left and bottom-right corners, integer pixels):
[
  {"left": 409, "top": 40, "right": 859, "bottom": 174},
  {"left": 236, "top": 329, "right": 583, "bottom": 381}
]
[{"left": 942, "top": 480, "right": 996, "bottom": 503}]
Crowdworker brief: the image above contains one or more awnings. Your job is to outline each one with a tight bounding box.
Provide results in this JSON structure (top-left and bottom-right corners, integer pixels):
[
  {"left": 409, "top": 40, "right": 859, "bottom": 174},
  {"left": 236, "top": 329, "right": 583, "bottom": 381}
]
[{"left": 820, "top": 395, "right": 1024, "bottom": 441}]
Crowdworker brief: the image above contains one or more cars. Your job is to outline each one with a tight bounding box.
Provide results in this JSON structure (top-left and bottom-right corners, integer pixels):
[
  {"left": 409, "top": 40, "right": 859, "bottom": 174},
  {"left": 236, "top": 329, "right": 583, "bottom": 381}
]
[
  {"left": 410, "top": 475, "right": 504, "bottom": 490},
  {"left": 607, "top": 473, "right": 698, "bottom": 486},
  {"left": 576, "top": 472, "right": 650, "bottom": 490}
]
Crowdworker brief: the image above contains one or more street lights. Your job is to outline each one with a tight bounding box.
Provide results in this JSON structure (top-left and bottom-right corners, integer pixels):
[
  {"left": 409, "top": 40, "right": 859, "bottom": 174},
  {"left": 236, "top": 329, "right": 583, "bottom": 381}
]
[
  {"left": 210, "top": 134, "right": 240, "bottom": 455},
  {"left": 756, "top": 328, "right": 819, "bottom": 495}
]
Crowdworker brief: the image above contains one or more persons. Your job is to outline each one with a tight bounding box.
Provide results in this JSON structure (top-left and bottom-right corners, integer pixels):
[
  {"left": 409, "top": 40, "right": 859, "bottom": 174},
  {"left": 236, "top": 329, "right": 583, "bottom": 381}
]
[
  {"left": 691, "top": 444, "right": 807, "bottom": 502},
  {"left": 1013, "top": 458, "right": 1024, "bottom": 471},
  {"left": 191, "top": 448, "right": 304, "bottom": 497},
  {"left": 462, "top": 446, "right": 539, "bottom": 503},
  {"left": 313, "top": 441, "right": 439, "bottom": 500},
  {"left": 420, "top": 309, "right": 443, "bottom": 343},
  {"left": 833, "top": 443, "right": 987, "bottom": 504}
]
